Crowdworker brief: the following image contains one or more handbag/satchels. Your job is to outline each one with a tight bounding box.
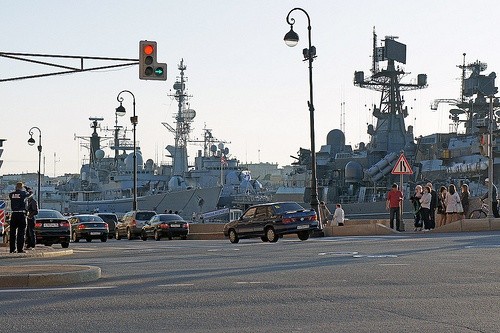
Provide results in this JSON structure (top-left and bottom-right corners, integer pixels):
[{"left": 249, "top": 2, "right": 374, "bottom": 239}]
[
  {"left": 322, "top": 218, "right": 328, "bottom": 224},
  {"left": 456, "top": 202, "right": 464, "bottom": 213},
  {"left": 330, "top": 218, "right": 339, "bottom": 227}
]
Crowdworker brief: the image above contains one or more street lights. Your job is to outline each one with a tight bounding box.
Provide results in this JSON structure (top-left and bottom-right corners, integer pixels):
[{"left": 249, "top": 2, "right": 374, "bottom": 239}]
[
  {"left": 115, "top": 89, "right": 138, "bottom": 211},
  {"left": 27, "top": 126, "right": 42, "bottom": 210},
  {"left": 283, "top": 6, "right": 325, "bottom": 238}
]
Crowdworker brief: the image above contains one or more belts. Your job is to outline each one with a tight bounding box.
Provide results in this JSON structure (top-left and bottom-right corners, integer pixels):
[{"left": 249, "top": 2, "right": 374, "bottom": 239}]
[{"left": 12, "top": 211, "right": 23, "bottom": 212}]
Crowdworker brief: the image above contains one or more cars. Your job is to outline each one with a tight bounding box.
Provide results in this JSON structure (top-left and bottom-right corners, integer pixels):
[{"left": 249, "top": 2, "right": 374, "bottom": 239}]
[
  {"left": 66, "top": 214, "right": 110, "bottom": 242},
  {"left": 33, "top": 208, "right": 72, "bottom": 249},
  {"left": 223, "top": 201, "right": 321, "bottom": 245},
  {"left": 92, "top": 212, "right": 119, "bottom": 240},
  {"left": 113, "top": 209, "right": 158, "bottom": 241},
  {"left": 140, "top": 213, "right": 190, "bottom": 242}
]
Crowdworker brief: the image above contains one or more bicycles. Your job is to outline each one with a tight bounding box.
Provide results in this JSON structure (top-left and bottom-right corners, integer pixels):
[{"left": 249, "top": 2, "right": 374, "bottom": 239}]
[{"left": 466, "top": 200, "right": 499, "bottom": 219}]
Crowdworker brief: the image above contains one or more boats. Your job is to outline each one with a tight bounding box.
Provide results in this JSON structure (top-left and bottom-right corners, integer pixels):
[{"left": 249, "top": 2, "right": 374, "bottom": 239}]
[{"left": 2, "top": 25, "right": 500, "bottom": 222}]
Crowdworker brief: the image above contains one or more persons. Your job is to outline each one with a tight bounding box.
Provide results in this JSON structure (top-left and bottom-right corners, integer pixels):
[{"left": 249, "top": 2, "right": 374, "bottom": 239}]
[
  {"left": 332, "top": 204, "right": 345, "bottom": 226},
  {"left": 460, "top": 183, "right": 470, "bottom": 224},
  {"left": 410, "top": 185, "right": 425, "bottom": 232},
  {"left": 24, "top": 191, "right": 39, "bottom": 250},
  {"left": 419, "top": 185, "right": 432, "bottom": 231},
  {"left": 444, "top": 184, "right": 460, "bottom": 224},
  {"left": 8, "top": 182, "right": 34, "bottom": 253},
  {"left": 436, "top": 186, "right": 448, "bottom": 226},
  {"left": 426, "top": 182, "right": 438, "bottom": 230},
  {"left": 386, "top": 183, "right": 404, "bottom": 232},
  {"left": 479, "top": 178, "right": 500, "bottom": 218},
  {"left": 319, "top": 201, "right": 333, "bottom": 229}
]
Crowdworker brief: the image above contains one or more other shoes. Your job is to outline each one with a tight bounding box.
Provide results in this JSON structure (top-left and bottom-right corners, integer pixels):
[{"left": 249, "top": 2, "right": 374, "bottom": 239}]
[
  {"left": 422, "top": 228, "right": 433, "bottom": 232},
  {"left": 399, "top": 227, "right": 405, "bottom": 231},
  {"left": 11, "top": 250, "right": 26, "bottom": 253},
  {"left": 413, "top": 227, "right": 422, "bottom": 231},
  {"left": 24, "top": 246, "right": 33, "bottom": 250}
]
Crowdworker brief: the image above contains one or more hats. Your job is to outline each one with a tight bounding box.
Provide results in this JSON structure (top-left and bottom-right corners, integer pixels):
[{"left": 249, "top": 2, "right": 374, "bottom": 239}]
[{"left": 485, "top": 178, "right": 489, "bottom": 182}]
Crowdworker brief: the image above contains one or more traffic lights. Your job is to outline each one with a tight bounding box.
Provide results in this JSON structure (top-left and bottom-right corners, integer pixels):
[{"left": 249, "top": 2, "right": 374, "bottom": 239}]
[{"left": 138, "top": 39, "right": 168, "bottom": 81}]
